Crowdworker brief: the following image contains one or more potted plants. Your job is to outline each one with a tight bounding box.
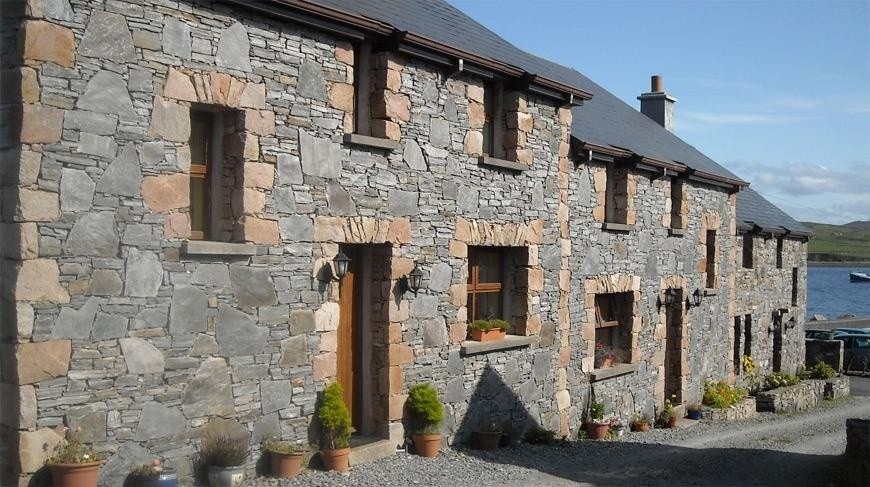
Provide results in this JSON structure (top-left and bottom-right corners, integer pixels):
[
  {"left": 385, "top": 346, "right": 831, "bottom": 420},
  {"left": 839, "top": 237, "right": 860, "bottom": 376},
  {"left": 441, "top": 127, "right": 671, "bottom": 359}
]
[
  {"left": 316, "top": 380, "right": 356, "bottom": 473},
  {"left": 193, "top": 432, "right": 253, "bottom": 486},
  {"left": 261, "top": 431, "right": 304, "bottom": 479},
  {"left": 629, "top": 410, "right": 651, "bottom": 432},
  {"left": 467, "top": 319, "right": 512, "bottom": 341},
  {"left": 585, "top": 401, "right": 609, "bottom": 440},
  {"left": 406, "top": 382, "right": 443, "bottom": 458}
]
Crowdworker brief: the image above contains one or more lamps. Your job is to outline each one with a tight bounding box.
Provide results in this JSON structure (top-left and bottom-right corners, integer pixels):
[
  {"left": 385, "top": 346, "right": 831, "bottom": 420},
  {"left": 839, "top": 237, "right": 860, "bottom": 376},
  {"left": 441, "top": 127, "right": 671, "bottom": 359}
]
[
  {"left": 784, "top": 315, "right": 796, "bottom": 335},
  {"left": 399, "top": 261, "right": 422, "bottom": 298},
  {"left": 685, "top": 288, "right": 703, "bottom": 315},
  {"left": 768, "top": 317, "right": 781, "bottom": 337},
  {"left": 655, "top": 286, "right": 677, "bottom": 314},
  {"left": 325, "top": 248, "right": 352, "bottom": 298}
]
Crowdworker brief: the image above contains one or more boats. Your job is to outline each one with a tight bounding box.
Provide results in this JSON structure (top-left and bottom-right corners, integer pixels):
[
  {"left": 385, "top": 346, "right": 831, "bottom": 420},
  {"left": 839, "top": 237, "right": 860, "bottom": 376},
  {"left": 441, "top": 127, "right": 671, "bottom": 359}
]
[{"left": 850, "top": 272, "right": 870, "bottom": 282}]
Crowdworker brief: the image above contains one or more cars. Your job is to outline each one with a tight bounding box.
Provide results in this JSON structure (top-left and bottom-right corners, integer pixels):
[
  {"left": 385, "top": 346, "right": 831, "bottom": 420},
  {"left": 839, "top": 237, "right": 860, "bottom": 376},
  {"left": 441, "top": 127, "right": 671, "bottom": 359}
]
[{"left": 803, "top": 328, "right": 870, "bottom": 371}]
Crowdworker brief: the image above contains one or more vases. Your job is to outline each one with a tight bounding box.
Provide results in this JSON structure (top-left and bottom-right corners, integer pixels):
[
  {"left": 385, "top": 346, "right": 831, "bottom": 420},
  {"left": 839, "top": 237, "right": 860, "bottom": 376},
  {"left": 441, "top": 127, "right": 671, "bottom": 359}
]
[
  {"left": 661, "top": 417, "right": 677, "bottom": 428},
  {"left": 130, "top": 468, "right": 179, "bottom": 486},
  {"left": 472, "top": 431, "right": 501, "bottom": 450},
  {"left": 613, "top": 428, "right": 626, "bottom": 437},
  {"left": 43, "top": 459, "right": 105, "bottom": 486},
  {"left": 601, "top": 356, "right": 614, "bottom": 365}
]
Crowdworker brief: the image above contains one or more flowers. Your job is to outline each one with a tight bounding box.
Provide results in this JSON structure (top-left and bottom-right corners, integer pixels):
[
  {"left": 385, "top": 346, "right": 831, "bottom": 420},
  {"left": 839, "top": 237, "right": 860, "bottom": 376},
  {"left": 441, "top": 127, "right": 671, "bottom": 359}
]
[
  {"left": 131, "top": 457, "right": 162, "bottom": 478},
  {"left": 658, "top": 394, "right": 677, "bottom": 423},
  {"left": 42, "top": 424, "right": 100, "bottom": 463},
  {"left": 610, "top": 416, "right": 623, "bottom": 431},
  {"left": 596, "top": 341, "right": 628, "bottom": 367},
  {"left": 744, "top": 353, "right": 756, "bottom": 374}
]
